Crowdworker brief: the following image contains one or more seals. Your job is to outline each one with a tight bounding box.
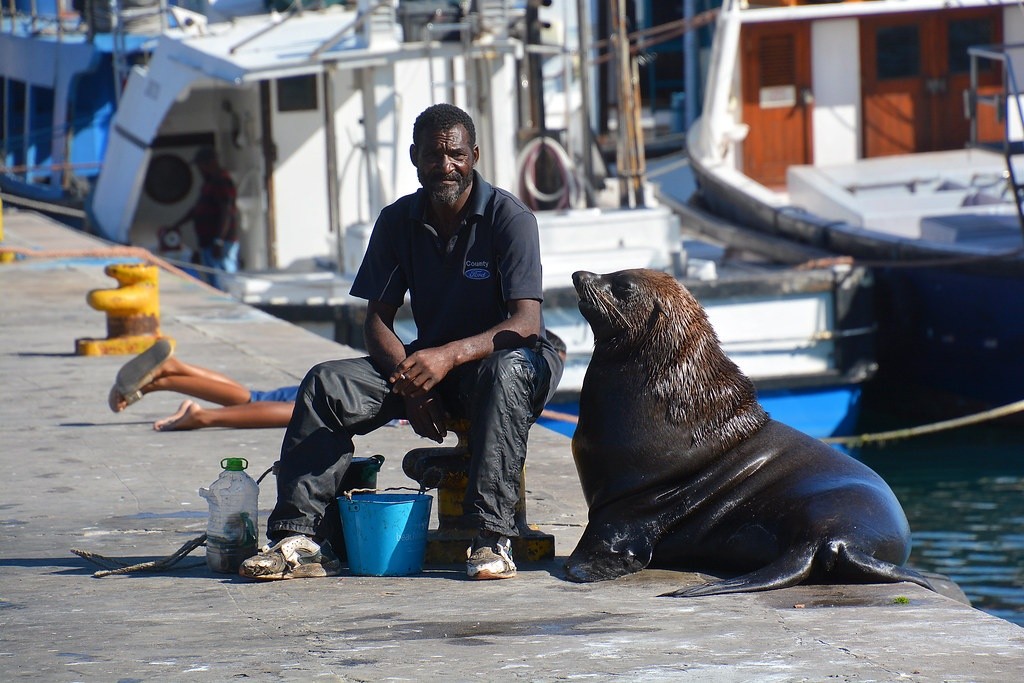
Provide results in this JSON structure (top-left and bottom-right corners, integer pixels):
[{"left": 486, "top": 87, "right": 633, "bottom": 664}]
[{"left": 550, "top": 268, "right": 939, "bottom": 598}]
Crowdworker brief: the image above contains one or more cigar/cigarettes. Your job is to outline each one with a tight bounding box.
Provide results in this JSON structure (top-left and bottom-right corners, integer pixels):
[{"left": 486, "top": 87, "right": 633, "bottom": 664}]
[{"left": 400, "top": 373, "right": 405, "bottom": 380}]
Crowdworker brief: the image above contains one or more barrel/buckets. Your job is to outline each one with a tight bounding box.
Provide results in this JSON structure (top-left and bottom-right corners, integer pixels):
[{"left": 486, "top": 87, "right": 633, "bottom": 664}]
[
  {"left": 336, "top": 488, "right": 433, "bottom": 577},
  {"left": 275, "top": 454, "right": 386, "bottom": 567}
]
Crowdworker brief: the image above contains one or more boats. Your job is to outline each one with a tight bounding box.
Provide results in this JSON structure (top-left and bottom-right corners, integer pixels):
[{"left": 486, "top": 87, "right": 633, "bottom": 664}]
[{"left": 0, "top": 0, "right": 1022, "bottom": 457}]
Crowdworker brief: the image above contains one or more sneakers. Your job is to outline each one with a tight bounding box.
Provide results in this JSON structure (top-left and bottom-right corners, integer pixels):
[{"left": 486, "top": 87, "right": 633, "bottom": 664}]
[
  {"left": 466, "top": 536, "right": 515, "bottom": 579},
  {"left": 238, "top": 533, "right": 340, "bottom": 580}
]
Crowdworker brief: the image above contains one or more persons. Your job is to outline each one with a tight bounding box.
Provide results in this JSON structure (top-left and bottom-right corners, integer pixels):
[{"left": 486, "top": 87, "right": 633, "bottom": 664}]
[
  {"left": 238, "top": 102, "right": 567, "bottom": 581},
  {"left": 107, "top": 337, "right": 410, "bottom": 433}
]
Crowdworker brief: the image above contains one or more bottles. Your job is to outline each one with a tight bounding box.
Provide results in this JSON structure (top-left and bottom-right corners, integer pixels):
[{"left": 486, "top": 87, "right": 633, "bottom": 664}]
[{"left": 198, "top": 457, "right": 260, "bottom": 573}]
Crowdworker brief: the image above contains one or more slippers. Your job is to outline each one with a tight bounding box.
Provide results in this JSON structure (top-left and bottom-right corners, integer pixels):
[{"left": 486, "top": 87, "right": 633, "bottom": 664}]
[{"left": 110, "top": 342, "right": 173, "bottom": 412}]
